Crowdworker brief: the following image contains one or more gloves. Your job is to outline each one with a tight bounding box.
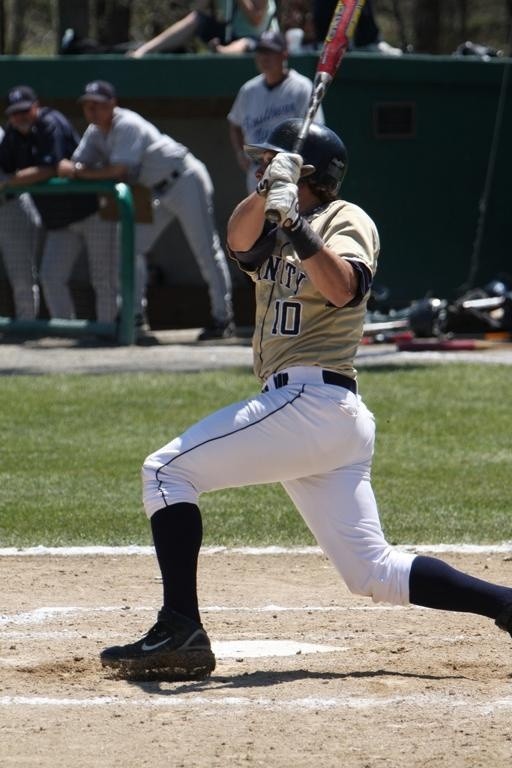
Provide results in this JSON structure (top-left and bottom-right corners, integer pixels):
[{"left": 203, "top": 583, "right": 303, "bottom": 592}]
[
  {"left": 255, "top": 153, "right": 315, "bottom": 195},
  {"left": 262, "top": 179, "right": 303, "bottom": 233}
]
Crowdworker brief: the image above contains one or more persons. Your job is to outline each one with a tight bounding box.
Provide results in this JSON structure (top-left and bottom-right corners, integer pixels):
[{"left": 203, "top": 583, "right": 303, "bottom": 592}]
[
  {"left": 1, "top": 84, "right": 119, "bottom": 322},
  {"left": 56, "top": 77, "right": 237, "bottom": 341},
  {"left": 1, "top": 124, "right": 43, "bottom": 320},
  {"left": 126, "top": 0, "right": 287, "bottom": 58},
  {"left": 227, "top": 33, "right": 327, "bottom": 196},
  {"left": 97, "top": 116, "right": 511, "bottom": 668}
]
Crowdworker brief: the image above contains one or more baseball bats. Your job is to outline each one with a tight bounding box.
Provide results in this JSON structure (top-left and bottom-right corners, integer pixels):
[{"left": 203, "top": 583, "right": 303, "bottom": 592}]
[{"left": 266, "top": 0, "right": 363, "bottom": 224}]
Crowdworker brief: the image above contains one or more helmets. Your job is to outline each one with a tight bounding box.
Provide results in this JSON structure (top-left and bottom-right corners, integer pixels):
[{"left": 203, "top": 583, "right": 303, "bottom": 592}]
[{"left": 242, "top": 117, "right": 350, "bottom": 201}]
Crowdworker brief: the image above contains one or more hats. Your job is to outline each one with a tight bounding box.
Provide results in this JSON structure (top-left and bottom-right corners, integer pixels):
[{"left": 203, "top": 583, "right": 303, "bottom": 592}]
[
  {"left": 5, "top": 86, "right": 36, "bottom": 114},
  {"left": 254, "top": 30, "right": 286, "bottom": 55},
  {"left": 79, "top": 80, "right": 115, "bottom": 103}
]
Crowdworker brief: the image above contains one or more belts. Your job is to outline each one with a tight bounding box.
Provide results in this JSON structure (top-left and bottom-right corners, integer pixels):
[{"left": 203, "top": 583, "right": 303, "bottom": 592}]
[
  {"left": 149, "top": 167, "right": 185, "bottom": 197},
  {"left": 261, "top": 371, "right": 357, "bottom": 395}
]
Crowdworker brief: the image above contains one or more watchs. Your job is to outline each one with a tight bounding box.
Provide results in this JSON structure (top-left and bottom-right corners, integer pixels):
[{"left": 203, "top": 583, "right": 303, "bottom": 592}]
[{"left": 73, "top": 159, "right": 87, "bottom": 176}]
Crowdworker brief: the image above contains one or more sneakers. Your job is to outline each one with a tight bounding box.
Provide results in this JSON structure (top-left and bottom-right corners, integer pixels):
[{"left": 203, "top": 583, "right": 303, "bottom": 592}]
[
  {"left": 197, "top": 315, "right": 236, "bottom": 341},
  {"left": 100, "top": 624, "right": 216, "bottom": 678},
  {"left": 114, "top": 310, "right": 151, "bottom": 332}
]
